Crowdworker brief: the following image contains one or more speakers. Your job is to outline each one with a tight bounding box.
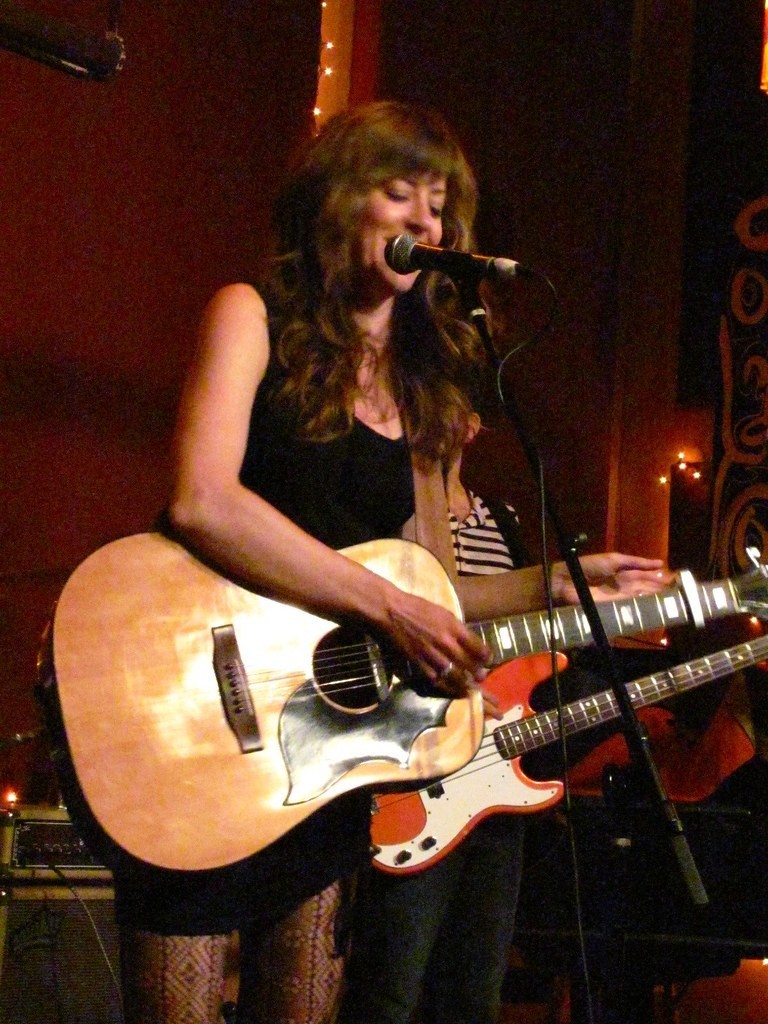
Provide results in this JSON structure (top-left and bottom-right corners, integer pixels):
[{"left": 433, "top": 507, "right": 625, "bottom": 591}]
[{"left": 0, "top": 881, "right": 132, "bottom": 1024}]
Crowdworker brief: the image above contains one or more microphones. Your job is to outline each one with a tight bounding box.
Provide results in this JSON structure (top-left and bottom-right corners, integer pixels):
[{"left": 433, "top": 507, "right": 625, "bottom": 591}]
[{"left": 384, "top": 234, "right": 529, "bottom": 279}]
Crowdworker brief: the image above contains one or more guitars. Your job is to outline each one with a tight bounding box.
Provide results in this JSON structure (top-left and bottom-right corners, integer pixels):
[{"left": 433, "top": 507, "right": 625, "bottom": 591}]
[
  {"left": 367, "top": 633, "right": 766, "bottom": 878},
  {"left": 37, "top": 529, "right": 767, "bottom": 895}
]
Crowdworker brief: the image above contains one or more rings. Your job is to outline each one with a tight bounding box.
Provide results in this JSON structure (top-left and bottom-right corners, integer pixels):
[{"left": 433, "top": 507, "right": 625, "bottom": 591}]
[{"left": 441, "top": 662, "right": 452, "bottom": 678}]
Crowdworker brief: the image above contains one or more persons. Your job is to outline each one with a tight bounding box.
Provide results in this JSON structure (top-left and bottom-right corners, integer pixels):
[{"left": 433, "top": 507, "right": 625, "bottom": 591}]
[
  {"left": 112, "top": 100, "right": 678, "bottom": 1024},
  {"left": 339, "top": 380, "right": 529, "bottom": 1024}
]
